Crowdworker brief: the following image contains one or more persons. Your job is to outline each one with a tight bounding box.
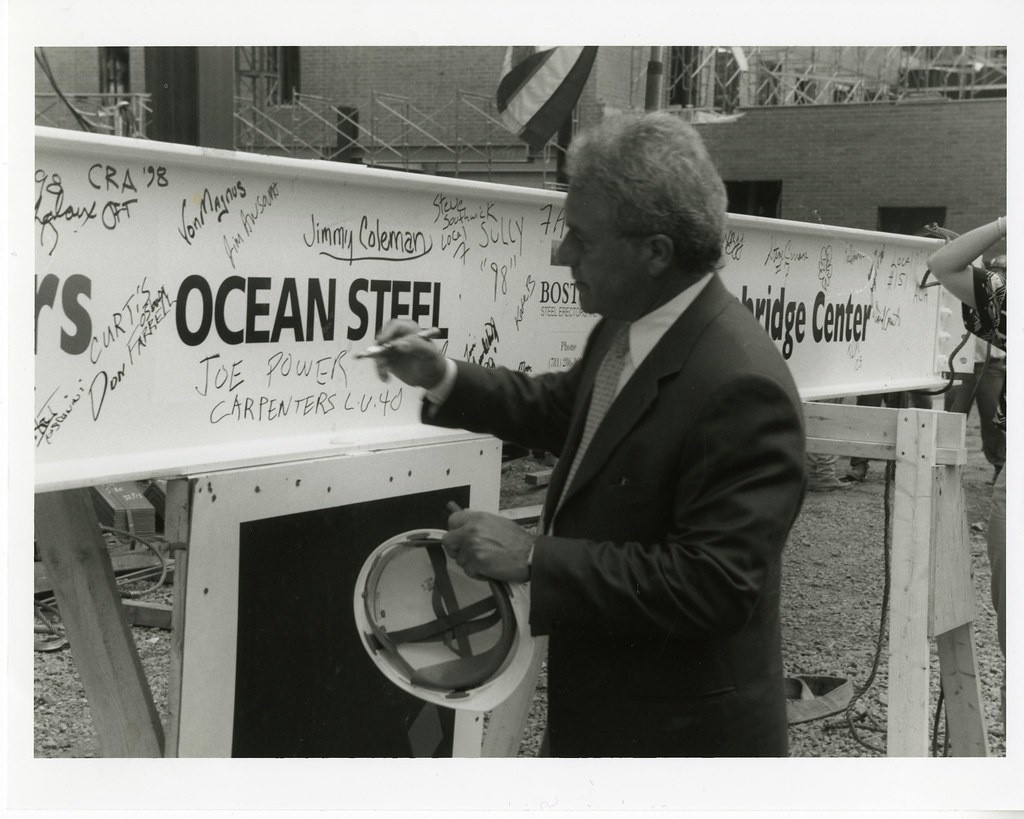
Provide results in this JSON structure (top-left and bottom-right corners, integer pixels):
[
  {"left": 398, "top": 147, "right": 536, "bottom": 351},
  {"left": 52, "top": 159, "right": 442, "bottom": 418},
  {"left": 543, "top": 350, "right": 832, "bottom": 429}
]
[
  {"left": 804, "top": 335, "right": 1006, "bottom": 491},
  {"left": 927, "top": 213, "right": 1006, "bottom": 753},
  {"left": 372, "top": 111, "right": 807, "bottom": 758}
]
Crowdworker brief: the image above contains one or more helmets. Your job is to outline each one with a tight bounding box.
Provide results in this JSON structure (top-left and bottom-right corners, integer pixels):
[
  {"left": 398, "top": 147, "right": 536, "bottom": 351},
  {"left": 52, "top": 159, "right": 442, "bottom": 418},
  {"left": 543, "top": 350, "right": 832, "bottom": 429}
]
[{"left": 352, "top": 528, "right": 536, "bottom": 713}]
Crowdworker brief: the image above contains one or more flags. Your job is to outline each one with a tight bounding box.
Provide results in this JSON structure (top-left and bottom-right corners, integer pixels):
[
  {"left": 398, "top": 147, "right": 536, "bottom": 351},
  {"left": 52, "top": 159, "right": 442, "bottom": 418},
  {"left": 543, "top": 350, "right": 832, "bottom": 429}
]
[{"left": 496, "top": 46, "right": 601, "bottom": 159}]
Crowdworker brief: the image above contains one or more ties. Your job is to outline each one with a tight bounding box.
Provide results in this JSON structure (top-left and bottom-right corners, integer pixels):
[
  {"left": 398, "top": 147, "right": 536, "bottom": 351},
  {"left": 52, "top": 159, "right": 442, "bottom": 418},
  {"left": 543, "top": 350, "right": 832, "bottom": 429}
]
[{"left": 551, "top": 316, "right": 633, "bottom": 523}]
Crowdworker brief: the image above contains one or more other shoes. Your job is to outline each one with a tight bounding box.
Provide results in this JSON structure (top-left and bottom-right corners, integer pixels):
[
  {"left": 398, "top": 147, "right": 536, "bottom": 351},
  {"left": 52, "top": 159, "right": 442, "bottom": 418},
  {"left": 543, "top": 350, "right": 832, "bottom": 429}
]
[
  {"left": 832, "top": 476, "right": 854, "bottom": 491},
  {"left": 845, "top": 468, "right": 868, "bottom": 480}
]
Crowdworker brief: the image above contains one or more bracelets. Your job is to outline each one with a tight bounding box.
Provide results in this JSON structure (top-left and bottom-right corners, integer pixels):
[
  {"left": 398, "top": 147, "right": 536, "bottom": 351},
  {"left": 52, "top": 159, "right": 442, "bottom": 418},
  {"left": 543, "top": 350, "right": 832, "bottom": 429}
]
[
  {"left": 997, "top": 216, "right": 1002, "bottom": 236},
  {"left": 527, "top": 542, "right": 534, "bottom": 573}
]
